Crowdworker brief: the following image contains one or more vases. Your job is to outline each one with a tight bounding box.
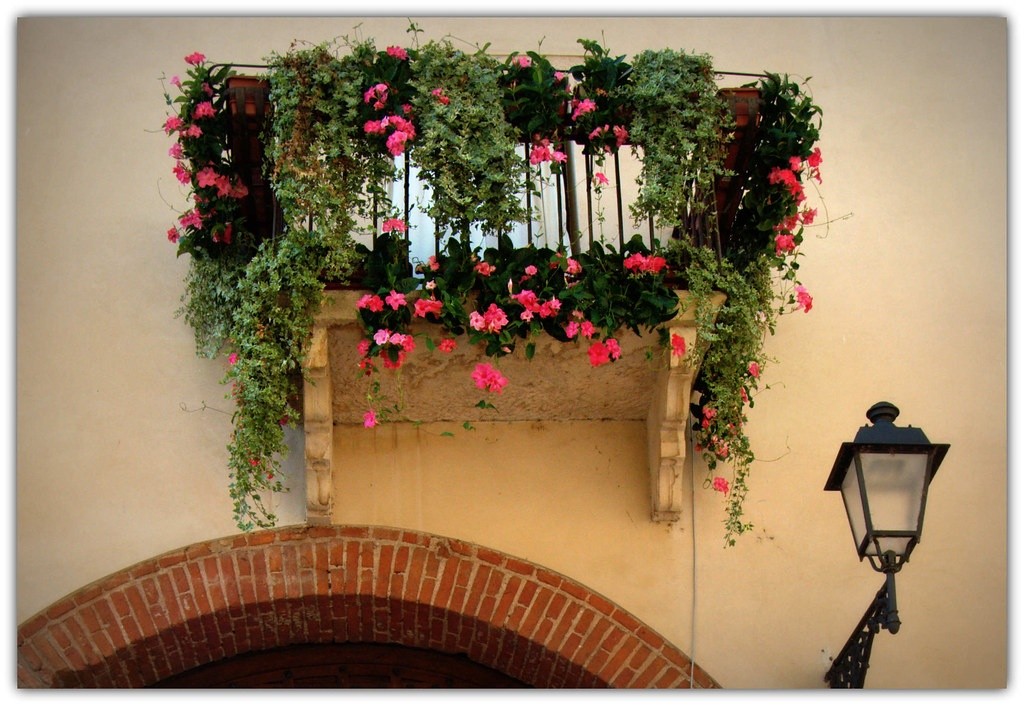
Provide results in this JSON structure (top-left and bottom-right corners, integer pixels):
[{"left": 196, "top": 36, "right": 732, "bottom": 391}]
[{"left": 227, "top": 75, "right": 761, "bottom": 290}]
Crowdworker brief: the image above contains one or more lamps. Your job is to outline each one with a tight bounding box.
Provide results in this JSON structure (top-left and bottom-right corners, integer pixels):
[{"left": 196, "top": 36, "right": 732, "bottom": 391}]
[{"left": 823, "top": 400, "right": 950, "bottom": 689}]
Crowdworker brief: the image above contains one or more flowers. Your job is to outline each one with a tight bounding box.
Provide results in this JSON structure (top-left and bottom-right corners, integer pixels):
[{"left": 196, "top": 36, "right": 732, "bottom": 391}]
[{"left": 147, "top": 15, "right": 854, "bottom": 554}]
